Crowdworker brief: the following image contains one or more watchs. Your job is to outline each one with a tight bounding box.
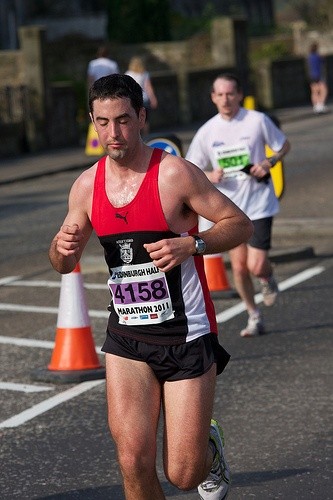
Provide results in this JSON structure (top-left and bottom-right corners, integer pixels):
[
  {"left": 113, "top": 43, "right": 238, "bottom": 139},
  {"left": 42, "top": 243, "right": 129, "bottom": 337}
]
[{"left": 189, "top": 235, "right": 206, "bottom": 256}]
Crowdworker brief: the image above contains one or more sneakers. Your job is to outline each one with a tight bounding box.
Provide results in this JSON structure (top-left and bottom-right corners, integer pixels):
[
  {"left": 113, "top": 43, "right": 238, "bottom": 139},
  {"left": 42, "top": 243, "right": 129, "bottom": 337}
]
[
  {"left": 260, "top": 262, "right": 279, "bottom": 307},
  {"left": 240, "top": 309, "right": 262, "bottom": 336},
  {"left": 197, "top": 419, "right": 230, "bottom": 500}
]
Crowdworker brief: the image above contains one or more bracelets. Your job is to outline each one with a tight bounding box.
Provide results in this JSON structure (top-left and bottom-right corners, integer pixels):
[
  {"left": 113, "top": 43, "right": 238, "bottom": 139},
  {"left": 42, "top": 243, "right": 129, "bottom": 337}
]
[{"left": 266, "top": 156, "right": 277, "bottom": 167}]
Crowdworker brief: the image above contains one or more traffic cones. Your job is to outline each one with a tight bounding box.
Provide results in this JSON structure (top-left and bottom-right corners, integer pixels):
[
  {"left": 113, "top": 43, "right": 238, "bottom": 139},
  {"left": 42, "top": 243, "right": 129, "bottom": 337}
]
[
  {"left": 197, "top": 215, "right": 240, "bottom": 298},
  {"left": 30, "top": 260, "right": 106, "bottom": 383}
]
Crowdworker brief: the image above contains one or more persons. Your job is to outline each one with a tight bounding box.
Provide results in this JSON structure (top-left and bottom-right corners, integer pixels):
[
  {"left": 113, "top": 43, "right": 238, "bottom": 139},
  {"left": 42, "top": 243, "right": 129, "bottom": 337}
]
[
  {"left": 88, "top": 45, "right": 117, "bottom": 91},
  {"left": 48, "top": 74, "right": 253, "bottom": 500},
  {"left": 124, "top": 57, "right": 157, "bottom": 136},
  {"left": 307, "top": 41, "right": 327, "bottom": 112},
  {"left": 184, "top": 73, "right": 291, "bottom": 336}
]
